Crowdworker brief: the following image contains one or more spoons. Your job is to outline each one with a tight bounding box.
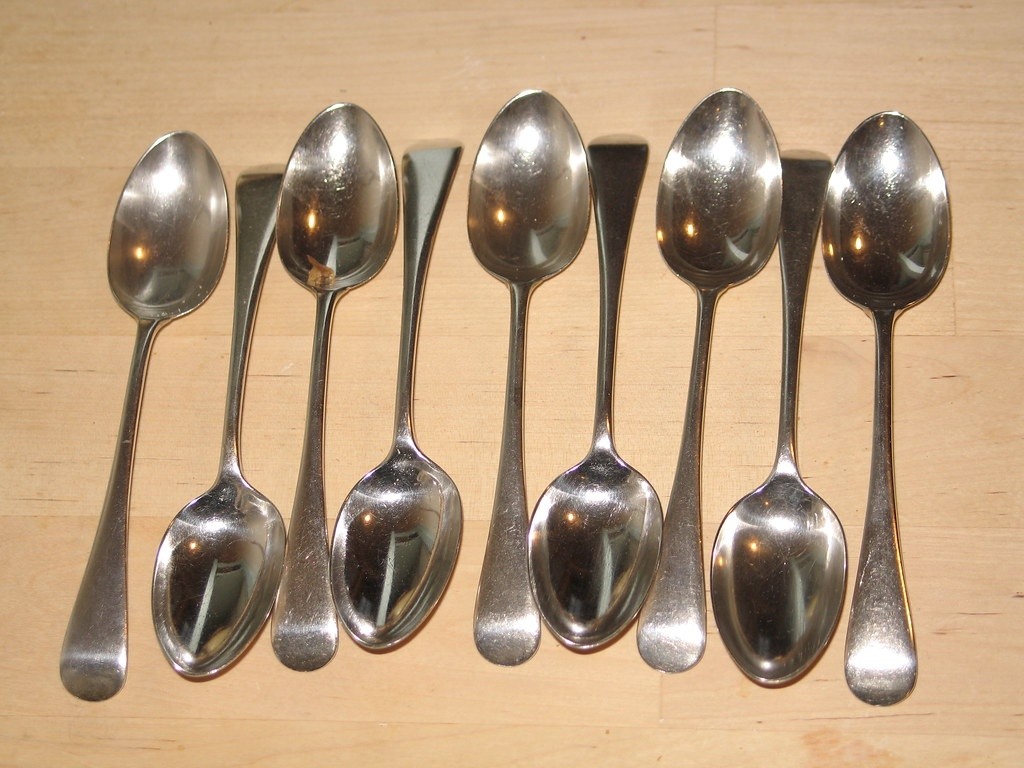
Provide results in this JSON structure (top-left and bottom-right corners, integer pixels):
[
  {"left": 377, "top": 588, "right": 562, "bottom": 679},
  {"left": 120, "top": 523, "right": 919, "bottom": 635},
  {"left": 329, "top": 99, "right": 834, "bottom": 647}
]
[
  {"left": 269, "top": 101, "right": 401, "bottom": 672},
  {"left": 467, "top": 89, "right": 592, "bottom": 667},
  {"left": 58, "top": 129, "right": 230, "bottom": 701},
  {"left": 636, "top": 87, "right": 786, "bottom": 671},
  {"left": 710, "top": 149, "right": 847, "bottom": 688},
  {"left": 526, "top": 131, "right": 666, "bottom": 654},
  {"left": 329, "top": 138, "right": 464, "bottom": 653},
  {"left": 151, "top": 164, "right": 287, "bottom": 679},
  {"left": 821, "top": 110, "right": 951, "bottom": 707}
]
